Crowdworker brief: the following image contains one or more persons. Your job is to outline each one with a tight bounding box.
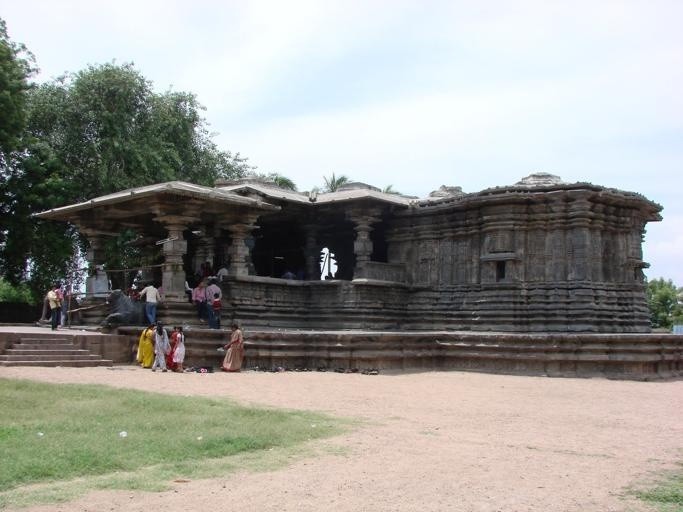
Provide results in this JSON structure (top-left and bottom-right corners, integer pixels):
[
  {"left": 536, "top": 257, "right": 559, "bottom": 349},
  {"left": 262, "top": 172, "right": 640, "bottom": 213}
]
[
  {"left": 206, "top": 278, "right": 223, "bottom": 329},
  {"left": 55, "top": 285, "right": 63, "bottom": 306},
  {"left": 210, "top": 292, "right": 222, "bottom": 328},
  {"left": 136, "top": 324, "right": 186, "bottom": 373},
  {"left": 48, "top": 286, "right": 62, "bottom": 331},
  {"left": 192, "top": 281, "right": 208, "bottom": 323},
  {"left": 222, "top": 325, "right": 245, "bottom": 372},
  {"left": 138, "top": 282, "right": 161, "bottom": 325}
]
